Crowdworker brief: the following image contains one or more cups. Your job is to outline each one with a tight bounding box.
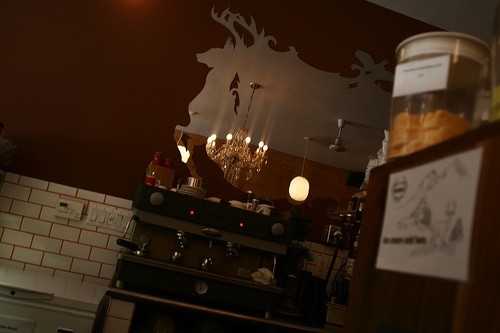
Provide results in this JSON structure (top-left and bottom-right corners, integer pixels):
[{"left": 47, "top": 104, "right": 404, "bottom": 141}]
[{"left": 144, "top": 174, "right": 160, "bottom": 187}]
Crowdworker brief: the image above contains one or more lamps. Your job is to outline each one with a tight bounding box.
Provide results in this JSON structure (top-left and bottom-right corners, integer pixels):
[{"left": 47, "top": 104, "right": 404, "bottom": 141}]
[
  {"left": 328, "top": 118, "right": 346, "bottom": 152},
  {"left": 287, "top": 136, "right": 313, "bottom": 202},
  {"left": 203, "top": 82, "right": 270, "bottom": 186}
]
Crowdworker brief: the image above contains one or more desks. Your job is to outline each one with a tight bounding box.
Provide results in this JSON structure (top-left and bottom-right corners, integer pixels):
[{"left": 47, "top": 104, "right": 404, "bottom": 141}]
[{"left": 0, "top": 265, "right": 111, "bottom": 333}]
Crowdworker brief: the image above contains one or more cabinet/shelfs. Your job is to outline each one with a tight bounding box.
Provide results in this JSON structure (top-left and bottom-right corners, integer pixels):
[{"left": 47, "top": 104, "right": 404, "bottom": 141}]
[{"left": 89, "top": 184, "right": 293, "bottom": 333}]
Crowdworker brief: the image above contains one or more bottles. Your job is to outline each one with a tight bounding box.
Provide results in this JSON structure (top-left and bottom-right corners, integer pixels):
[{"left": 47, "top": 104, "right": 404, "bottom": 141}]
[{"left": 386, "top": 31, "right": 494, "bottom": 163}]
